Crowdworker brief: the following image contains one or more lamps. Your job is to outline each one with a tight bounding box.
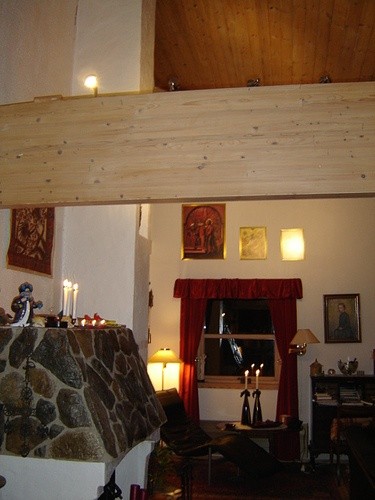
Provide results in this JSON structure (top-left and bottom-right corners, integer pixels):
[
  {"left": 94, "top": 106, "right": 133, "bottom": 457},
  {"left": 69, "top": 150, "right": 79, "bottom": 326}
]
[
  {"left": 280, "top": 228, "right": 304, "bottom": 261},
  {"left": 290, "top": 329, "right": 320, "bottom": 356},
  {"left": 148, "top": 348, "right": 182, "bottom": 391}
]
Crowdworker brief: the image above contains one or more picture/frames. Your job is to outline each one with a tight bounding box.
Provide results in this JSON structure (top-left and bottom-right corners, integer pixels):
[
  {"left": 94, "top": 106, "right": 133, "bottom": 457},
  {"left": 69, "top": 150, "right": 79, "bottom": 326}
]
[
  {"left": 239, "top": 226, "right": 267, "bottom": 260},
  {"left": 181, "top": 202, "right": 227, "bottom": 261},
  {"left": 323, "top": 293, "right": 362, "bottom": 343}
]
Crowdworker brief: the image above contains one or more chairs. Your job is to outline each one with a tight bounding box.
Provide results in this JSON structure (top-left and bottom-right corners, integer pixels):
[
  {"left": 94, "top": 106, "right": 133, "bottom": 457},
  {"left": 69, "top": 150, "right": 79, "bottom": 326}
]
[
  {"left": 330, "top": 403, "right": 375, "bottom": 486},
  {"left": 156, "top": 389, "right": 290, "bottom": 500}
]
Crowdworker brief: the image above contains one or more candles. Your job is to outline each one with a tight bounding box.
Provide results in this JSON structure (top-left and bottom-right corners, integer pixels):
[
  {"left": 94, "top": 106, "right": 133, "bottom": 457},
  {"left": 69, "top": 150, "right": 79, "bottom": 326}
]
[
  {"left": 245, "top": 370, "right": 249, "bottom": 390},
  {"left": 63, "top": 279, "right": 78, "bottom": 319},
  {"left": 256, "top": 370, "right": 260, "bottom": 389}
]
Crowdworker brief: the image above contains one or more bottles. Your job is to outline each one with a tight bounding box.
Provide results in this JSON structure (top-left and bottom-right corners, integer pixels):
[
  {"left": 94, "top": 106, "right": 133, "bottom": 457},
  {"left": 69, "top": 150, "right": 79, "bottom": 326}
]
[
  {"left": 252, "top": 389, "right": 262, "bottom": 425},
  {"left": 241, "top": 389, "right": 251, "bottom": 425}
]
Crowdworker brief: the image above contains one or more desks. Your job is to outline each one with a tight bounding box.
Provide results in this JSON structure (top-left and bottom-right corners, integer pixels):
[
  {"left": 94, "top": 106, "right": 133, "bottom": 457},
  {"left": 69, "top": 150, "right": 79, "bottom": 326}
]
[
  {"left": 200, "top": 420, "right": 287, "bottom": 458},
  {"left": 347, "top": 440, "right": 375, "bottom": 500}
]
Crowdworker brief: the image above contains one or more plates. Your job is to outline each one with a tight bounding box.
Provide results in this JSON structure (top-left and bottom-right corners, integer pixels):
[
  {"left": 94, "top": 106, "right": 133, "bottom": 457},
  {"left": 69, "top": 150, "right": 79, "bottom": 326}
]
[{"left": 249, "top": 422, "right": 283, "bottom": 428}]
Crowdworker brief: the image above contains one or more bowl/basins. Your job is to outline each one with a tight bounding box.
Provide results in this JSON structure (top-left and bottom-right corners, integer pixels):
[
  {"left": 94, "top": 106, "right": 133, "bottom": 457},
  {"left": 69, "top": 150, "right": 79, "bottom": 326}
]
[{"left": 338, "top": 360, "right": 358, "bottom": 375}]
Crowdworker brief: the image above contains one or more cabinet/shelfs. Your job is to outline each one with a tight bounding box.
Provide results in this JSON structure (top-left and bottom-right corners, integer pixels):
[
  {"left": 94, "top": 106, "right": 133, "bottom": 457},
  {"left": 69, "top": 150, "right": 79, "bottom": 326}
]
[{"left": 312, "top": 375, "right": 375, "bottom": 469}]
